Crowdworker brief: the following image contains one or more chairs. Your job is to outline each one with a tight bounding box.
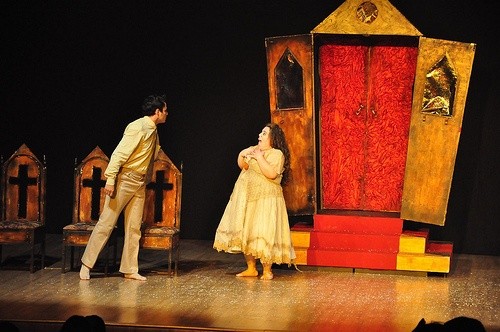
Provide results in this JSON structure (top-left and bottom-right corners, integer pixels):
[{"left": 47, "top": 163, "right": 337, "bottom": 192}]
[
  {"left": 0, "top": 143, "right": 47, "bottom": 273},
  {"left": 138, "top": 149, "right": 184, "bottom": 278},
  {"left": 61, "top": 145, "right": 117, "bottom": 278}
]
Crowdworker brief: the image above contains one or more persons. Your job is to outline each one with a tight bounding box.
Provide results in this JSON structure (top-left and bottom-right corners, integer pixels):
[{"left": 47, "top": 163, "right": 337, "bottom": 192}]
[
  {"left": 212, "top": 123, "right": 296, "bottom": 280},
  {"left": 79, "top": 95, "right": 168, "bottom": 281}
]
